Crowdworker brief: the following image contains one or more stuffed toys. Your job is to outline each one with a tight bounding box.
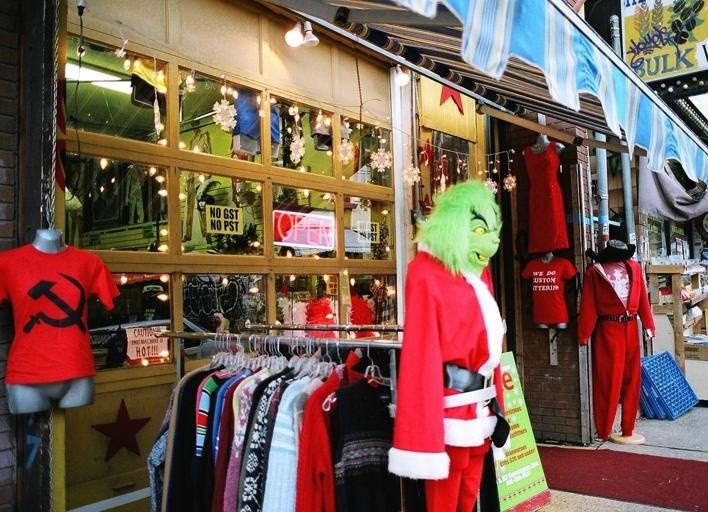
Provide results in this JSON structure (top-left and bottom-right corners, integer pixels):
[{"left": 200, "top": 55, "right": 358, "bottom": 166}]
[{"left": 388, "top": 179, "right": 505, "bottom": 512}]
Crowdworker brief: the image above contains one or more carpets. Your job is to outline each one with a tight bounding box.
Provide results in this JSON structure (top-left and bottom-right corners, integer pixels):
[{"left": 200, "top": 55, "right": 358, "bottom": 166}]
[{"left": 536, "top": 444, "right": 708, "bottom": 512}]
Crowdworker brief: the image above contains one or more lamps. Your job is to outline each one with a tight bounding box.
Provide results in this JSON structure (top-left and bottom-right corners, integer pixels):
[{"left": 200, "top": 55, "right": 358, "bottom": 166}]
[{"left": 285, "top": 16, "right": 320, "bottom": 48}]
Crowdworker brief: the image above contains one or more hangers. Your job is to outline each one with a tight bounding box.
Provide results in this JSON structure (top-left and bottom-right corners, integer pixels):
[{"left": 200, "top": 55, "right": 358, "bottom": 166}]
[{"left": 207, "top": 332, "right": 391, "bottom": 412}]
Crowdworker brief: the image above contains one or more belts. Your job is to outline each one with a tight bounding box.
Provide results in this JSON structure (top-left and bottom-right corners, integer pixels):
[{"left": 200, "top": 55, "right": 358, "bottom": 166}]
[{"left": 597, "top": 314, "right": 637, "bottom": 322}]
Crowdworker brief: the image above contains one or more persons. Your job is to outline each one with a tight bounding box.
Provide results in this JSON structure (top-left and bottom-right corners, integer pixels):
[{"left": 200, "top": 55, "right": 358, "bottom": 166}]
[
  {"left": 178, "top": 111, "right": 215, "bottom": 247},
  {"left": 308, "top": 106, "right": 349, "bottom": 152},
  {"left": 129, "top": 54, "right": 186, "bottom": 117},
  {"left": 93, "top": 159, "right": 119, "bottom": 221},
  {"left": 521, "top": 251, "right": 576, "bottom": 331},
  {"left": 125, "top": 160, "right": 144, "bottom": 225},
  {"left": 521, "top": 134, "right": 570, "bottom": 256},
  {"left": 0, "top": 227, "right": 122, "bottom": 415},
  {"left": 232, "top": 86, "right": 281, "bottom": 159},
  {"left": 577, "top": 240, "right": 656, "bottom": 445}
]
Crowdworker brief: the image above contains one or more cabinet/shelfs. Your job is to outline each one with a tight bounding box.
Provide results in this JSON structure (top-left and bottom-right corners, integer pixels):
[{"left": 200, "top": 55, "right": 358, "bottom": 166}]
[{"left": 646, "top": 261, "right": 708, "bottom": 315}]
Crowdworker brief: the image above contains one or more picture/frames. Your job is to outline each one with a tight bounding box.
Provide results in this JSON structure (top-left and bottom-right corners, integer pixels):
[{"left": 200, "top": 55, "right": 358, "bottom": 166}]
[{"left": 86, "top": 157, "right": 156, "bottom": 228}]
